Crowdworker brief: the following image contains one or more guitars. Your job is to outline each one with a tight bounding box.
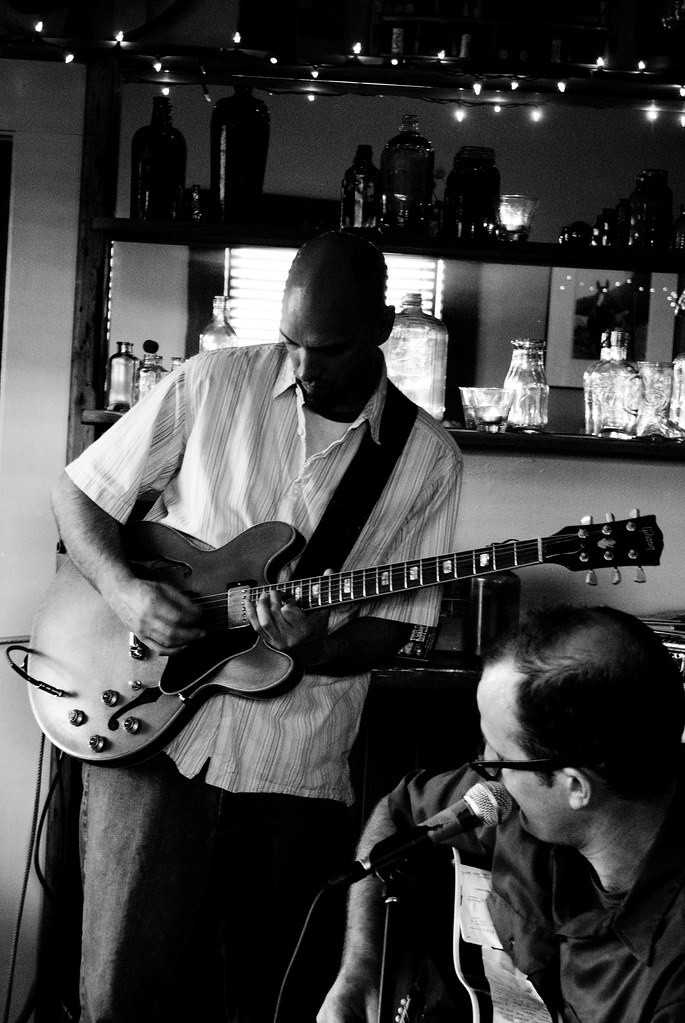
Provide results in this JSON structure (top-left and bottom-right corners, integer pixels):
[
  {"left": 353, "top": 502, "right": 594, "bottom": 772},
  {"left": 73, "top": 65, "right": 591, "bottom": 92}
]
[
  {"left": 24, "top": 504, "right": 668, "bottom": 767},
  {"left": 373, "top": 848, "right": 559, "bottom": 1023}
]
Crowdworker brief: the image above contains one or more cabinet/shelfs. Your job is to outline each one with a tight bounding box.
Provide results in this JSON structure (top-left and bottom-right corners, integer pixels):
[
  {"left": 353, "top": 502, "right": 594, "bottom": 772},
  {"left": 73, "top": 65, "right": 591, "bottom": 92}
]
[{"left": 82, "top": 205, "right": 685, "bottom": 464}]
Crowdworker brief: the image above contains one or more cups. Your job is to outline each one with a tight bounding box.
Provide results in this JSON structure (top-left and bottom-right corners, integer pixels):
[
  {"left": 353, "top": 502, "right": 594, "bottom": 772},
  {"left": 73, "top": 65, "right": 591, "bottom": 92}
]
[
  {"left": 458, "top": 386, "right": 515, "bottom": 434},
  {"left": 498, "top": 195, "right": 539, "bottom": 241},
  {"left": 504, "top": 339, "right": 549, "bottom": 434},
  {"left": 636, "top": 360, "right": 685, "bottom": 439}
]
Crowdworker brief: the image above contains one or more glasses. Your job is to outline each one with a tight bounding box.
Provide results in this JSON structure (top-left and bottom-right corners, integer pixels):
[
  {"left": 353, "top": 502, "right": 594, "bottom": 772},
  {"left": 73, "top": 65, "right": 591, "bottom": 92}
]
[{"left": 467, "top": 742, "right": 551, "bottom": 782}]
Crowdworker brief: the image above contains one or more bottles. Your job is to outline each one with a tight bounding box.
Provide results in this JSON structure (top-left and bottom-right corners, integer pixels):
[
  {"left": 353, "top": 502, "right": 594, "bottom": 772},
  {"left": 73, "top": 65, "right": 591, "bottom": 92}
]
[
  {"left": 442, "top": 146, "right": 501, "bottom": 250},
  {"left": 340, "top": 114, "right": 435, "bottom": 241},
  {"left": 592, "top": 169, "right": 685, "bottom": 246},
  {"left": 210, "top": 83, "right": 270, "bottom": 228},
  {"left": 200, "top": 296, "right": 242, "bottom": 352},
  {"left": 378, "top": 293, "right": 448, "bottom": 423},
  {"left": 130, "top": 97, "right": 187, "bottom": 228},
  {"left": 105, "top": 342, "right": 190, "bottom": 411},
  {"left": 583, "top": 329, "right": 645, "bottom": 440},
  {"left": 187, "top": 185, "right": 209, "bottom": 225}
]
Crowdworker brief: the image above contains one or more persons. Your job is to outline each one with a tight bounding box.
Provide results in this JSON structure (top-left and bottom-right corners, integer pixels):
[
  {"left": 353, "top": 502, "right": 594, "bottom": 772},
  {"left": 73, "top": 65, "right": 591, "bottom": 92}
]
[
  {"left": 315, "top": 604, "right": 685, "bottom": 1023},
  {"left": 48, "top": 228, "right": 463, "bottom": 1023}
]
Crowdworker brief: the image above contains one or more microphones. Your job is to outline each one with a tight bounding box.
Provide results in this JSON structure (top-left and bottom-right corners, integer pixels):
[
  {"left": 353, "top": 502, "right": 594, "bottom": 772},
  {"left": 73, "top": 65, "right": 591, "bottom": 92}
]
[{"left": 325, "top": 780, "right": 515, "bottom": 893}]
